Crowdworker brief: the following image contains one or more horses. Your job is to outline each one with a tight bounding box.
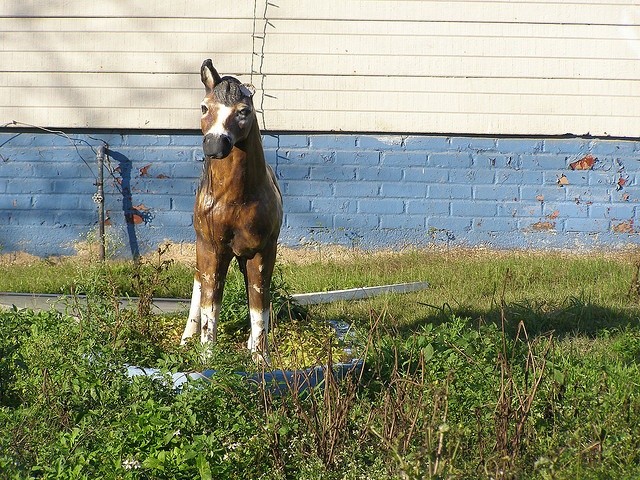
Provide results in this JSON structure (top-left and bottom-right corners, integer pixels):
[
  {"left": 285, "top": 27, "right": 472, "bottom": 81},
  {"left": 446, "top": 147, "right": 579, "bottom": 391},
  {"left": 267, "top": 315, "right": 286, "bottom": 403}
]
[{"left": 180, "top": 58, "right": 283, "bottom": 365}]
